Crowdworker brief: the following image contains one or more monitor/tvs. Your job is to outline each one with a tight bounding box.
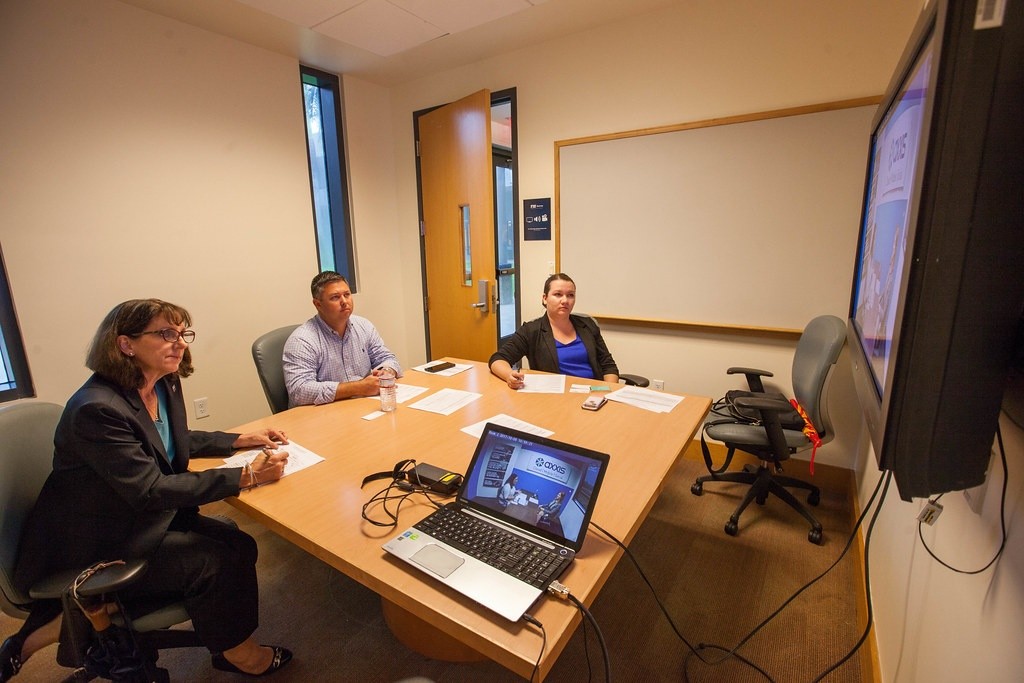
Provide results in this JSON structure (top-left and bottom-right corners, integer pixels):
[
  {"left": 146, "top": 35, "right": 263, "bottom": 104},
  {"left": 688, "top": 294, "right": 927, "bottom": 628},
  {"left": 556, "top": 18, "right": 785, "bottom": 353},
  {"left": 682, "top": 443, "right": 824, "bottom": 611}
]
[{"left": 846, "top": 0, "right": 1024, "bottom": 501}]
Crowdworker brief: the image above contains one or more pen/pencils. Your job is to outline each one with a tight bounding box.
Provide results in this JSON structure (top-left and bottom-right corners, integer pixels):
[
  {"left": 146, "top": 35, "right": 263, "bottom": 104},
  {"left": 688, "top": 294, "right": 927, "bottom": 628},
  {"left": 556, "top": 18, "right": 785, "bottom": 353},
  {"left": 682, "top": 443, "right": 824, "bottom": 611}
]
[
  {"left": 262, "top": 448, "right": 272, "bottom": 457},
  {"left": 517, "top": 367, "right": 520, "bottom": 380}
]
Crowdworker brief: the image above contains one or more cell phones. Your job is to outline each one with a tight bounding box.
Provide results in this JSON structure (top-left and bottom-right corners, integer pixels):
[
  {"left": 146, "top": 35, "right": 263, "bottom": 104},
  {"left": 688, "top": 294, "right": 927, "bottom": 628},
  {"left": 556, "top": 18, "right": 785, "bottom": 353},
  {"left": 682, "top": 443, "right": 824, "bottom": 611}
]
[
  {"left": 582, "top": 396, "right": 607, "bottom": 411},
  {"left": 425, "top": 362, "right": 455, "bottom": 373}
]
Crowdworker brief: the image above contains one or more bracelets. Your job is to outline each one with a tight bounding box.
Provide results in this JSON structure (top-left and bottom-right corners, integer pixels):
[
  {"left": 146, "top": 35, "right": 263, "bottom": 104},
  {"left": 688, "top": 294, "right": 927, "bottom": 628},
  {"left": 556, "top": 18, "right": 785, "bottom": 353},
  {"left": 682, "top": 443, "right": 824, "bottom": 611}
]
[{"left": 246, "top": 463, "right": 256, "bottom": 490}]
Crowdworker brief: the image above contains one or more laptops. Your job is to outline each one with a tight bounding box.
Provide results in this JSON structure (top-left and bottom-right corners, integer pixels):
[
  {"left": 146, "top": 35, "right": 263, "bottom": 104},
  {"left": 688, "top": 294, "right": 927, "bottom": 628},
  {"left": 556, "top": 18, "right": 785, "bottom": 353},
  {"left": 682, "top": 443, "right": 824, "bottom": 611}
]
[{"left": 382, "top": 422, "right": 609, "bottom": 622}]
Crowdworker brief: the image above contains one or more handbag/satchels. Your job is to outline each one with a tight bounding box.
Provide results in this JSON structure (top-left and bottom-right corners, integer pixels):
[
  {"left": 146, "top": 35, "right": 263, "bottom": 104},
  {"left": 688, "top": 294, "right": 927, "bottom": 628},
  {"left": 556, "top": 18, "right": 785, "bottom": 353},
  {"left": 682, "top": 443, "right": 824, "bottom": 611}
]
[{"left": 713, "top": 390, "right": 806, "bottom": 432}]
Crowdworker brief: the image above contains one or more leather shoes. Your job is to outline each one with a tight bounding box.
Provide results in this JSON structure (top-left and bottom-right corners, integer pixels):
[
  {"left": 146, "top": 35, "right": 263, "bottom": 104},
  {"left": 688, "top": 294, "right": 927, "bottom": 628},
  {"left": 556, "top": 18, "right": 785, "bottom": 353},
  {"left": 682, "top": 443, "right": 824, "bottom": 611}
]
[
  {"left": 212, "top": 644, "right": 293, "bottom": 678},
  {"left": 0, "top": 606, "right": 64, "bottom": 683}
]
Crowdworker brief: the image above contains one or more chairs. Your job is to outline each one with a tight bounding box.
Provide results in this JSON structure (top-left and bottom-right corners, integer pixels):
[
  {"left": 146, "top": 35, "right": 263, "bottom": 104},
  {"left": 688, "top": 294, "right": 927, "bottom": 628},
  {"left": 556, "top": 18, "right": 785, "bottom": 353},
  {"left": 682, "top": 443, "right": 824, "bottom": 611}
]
[
  {"left": 0, "top": 399, "right": 212, "bottom": 683},
  {"left": 251, "top": 323, "right": 304, "bottom": 414},
  {"left": 691, "top": 316, "right": 848, "bottom": 546}
]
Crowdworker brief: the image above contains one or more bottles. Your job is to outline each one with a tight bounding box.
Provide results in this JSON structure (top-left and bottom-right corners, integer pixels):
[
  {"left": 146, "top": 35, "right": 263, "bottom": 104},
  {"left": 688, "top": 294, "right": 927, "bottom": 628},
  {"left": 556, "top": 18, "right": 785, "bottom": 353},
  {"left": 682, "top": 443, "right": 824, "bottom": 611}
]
[{"left": 379, "top": 366, "right": 397, "bottom": 411}]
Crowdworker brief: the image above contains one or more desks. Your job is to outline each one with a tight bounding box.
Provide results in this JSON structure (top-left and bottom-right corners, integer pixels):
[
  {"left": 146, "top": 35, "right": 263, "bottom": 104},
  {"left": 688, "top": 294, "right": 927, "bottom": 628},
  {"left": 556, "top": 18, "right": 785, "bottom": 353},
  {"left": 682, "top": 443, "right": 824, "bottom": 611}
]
[{"left": 186, "top": 356, "right": 714, "bottom": 683}]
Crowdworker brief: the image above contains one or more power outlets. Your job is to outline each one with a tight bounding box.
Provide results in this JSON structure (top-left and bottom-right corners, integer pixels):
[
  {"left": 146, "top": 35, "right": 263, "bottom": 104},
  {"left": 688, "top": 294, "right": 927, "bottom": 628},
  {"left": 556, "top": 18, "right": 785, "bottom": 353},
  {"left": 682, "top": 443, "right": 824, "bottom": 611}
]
[{"left": 192, "top": 397, "right": 211, "bottom": 419}]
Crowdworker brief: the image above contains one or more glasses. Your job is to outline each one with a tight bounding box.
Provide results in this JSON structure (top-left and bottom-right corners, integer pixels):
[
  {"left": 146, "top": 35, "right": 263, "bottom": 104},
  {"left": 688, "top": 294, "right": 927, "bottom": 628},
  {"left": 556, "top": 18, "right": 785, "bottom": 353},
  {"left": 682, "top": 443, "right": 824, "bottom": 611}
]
[{"left": 126, "top": 329, "right": 195, "bottom": 343}]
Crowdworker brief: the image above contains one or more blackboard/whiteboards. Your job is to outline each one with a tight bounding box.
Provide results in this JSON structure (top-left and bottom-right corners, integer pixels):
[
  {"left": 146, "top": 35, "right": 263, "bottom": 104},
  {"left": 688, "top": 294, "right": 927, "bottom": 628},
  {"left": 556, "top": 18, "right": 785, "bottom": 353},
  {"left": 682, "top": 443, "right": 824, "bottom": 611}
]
[{"left": 553, "top": 93, "right": 885, "bottom": 335}]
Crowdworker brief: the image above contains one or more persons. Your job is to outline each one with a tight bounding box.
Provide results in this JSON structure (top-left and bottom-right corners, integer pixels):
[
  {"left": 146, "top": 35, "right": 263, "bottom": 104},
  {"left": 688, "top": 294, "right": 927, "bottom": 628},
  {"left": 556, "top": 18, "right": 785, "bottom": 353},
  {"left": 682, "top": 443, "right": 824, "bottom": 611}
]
[
  {"left": 489, "top": 274, "right": 620, "bottom": 390},
  {"left": 22, "top": 299, "right": 294, "bottom": 683},
  {"left": 499, "top": 474, "right": 519, "bottom": 507},
  {"left": 537, "top": 491, "right": 564, "bottom": 526},
  {"left": 284, "top": 271, "right": 401, "bottom": 408}
]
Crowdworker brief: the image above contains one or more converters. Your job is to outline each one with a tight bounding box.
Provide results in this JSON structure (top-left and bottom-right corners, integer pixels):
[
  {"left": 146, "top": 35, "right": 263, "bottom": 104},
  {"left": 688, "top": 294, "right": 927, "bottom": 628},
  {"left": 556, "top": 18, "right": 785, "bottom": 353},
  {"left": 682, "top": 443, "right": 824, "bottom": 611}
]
[{"left": 407, "top": 462, "right": 463, "bottom": 494}]
